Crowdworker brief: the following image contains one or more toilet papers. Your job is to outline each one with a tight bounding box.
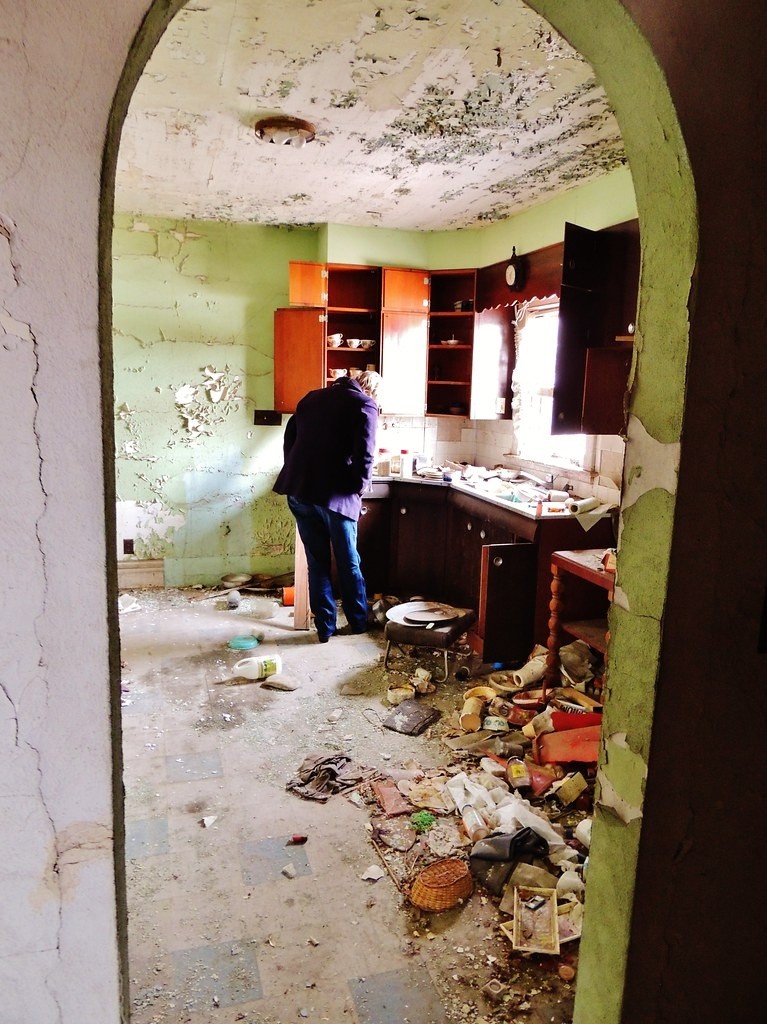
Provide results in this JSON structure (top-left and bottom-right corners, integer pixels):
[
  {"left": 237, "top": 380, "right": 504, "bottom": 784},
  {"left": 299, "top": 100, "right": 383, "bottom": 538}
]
[
  {"left": 513, "top": 657, "right": 545, "bottom": 688},
  {"left": 570, "top": 496, "right": 613, "bottom": 533}
]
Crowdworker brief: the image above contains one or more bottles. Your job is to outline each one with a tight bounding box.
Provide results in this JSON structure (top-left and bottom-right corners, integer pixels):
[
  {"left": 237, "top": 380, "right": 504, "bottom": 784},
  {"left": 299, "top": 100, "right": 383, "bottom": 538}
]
[
  {"left": 506, "top": 756, "right": 531, "bottom": 788},
  {"left": 231, "top": 655, "right": 282, "bottom": 680},
  {"left": 377, "top": 448, "right": 391, "bottom": 477},
  {"left": 227, "top": 590, "right": 240, "bottom": 611},
  {"left": 400, "top": 450, "right": 413, "bottom": 480},
  {"left": 462, "top": 804, "right": 488, "bottom": 842}
]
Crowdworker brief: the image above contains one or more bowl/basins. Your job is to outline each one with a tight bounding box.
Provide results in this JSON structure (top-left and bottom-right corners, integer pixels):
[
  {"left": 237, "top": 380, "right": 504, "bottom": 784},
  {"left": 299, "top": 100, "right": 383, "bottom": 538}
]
[
  {"left": 489, "top": 671, "right": 522, "bottom": 696},
  {"left": 227, "top": 635, "right": 263, "bottom": 650},
  {"left": 447, "top": 339, "right": 458, "bottom": 345},
  {"left": 221, "top": 573, "right": 252, "bottom": 589},
  {"left": 498, "top": 469, "right": 520, "bottom": 482},
  {"left": 463, "top": 687, "right": 497, "bottom": 710},
  {"left": 441, "top": 340, "right": 447, "bottom": 345}
]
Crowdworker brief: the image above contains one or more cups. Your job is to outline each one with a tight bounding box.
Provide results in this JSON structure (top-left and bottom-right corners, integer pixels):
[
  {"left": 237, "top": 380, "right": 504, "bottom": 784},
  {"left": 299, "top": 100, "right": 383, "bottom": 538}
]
[
  {"left": 347, "top": 339, "right": 362, "bottom": 348},
  {"left": 328, "top": 339, "right": 344, "bottom": 347},
  {"left": 328, "top": 333, "right": 343, "bottom": 339},
  {"left": 454, "top": 298, "right": 473, "bottom": 313},
  {"left": 361, "top": 340, "right": 376, "bottom": 348},
  {"left": 329, "top": 369, "right": 347, "bottom": 378},
  {"left": 459, "top": 698, "right": 485, "bottom": 733},
  {"left": 349, "top": 369, "right": 362, "bottom": 378}
]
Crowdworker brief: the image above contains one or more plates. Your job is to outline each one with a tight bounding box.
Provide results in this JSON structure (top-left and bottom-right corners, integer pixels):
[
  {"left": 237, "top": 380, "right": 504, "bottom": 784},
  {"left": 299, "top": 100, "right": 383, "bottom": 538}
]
[
  {"left": 406, "top": 610, "right": 458, "bottom": 622},
  {"left": 500, "top": 901, "right": 584, "bottom": 956},
  {"left": 386, "top": 601, "right": 453, "bottom": 626},
  {"left": 513, "top": 885, "right": 561, "bottom": 955}
]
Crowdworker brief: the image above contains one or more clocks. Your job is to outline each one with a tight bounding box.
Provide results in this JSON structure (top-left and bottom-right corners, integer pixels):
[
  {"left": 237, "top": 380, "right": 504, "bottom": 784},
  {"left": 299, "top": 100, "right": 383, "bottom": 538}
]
[{"left": 505, "top": 264, "right": 516, "bottom": 286}]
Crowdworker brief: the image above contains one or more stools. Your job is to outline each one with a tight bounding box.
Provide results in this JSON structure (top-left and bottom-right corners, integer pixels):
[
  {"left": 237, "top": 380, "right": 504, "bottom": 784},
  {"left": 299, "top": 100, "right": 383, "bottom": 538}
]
[{"left": 383, "top": 608, "right": 478, "bottom": 682}]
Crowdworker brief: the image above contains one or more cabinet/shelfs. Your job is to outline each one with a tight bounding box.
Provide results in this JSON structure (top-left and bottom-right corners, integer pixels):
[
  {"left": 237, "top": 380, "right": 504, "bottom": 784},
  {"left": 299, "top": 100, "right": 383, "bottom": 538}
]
[
  {"left": 304, "top": 484, "right": 622, "bottom": 706},
  {"left": 273, "top": 260, "right": 473, "bottom": 421},
  {"left": 549, "top": 220, "right": 643, "bottom": 436}
]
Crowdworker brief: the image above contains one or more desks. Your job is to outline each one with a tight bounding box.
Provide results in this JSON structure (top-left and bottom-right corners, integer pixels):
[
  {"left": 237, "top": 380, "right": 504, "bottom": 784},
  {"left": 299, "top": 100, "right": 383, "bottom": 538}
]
[{"left": 545, "top": 547, "right": 615, "bottom": 699}]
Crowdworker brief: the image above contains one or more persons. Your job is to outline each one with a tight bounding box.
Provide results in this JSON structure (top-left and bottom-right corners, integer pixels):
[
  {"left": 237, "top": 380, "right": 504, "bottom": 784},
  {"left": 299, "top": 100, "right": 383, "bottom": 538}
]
[{"left": 272, "top": 368, "right": 388, "bottom": 644}]
